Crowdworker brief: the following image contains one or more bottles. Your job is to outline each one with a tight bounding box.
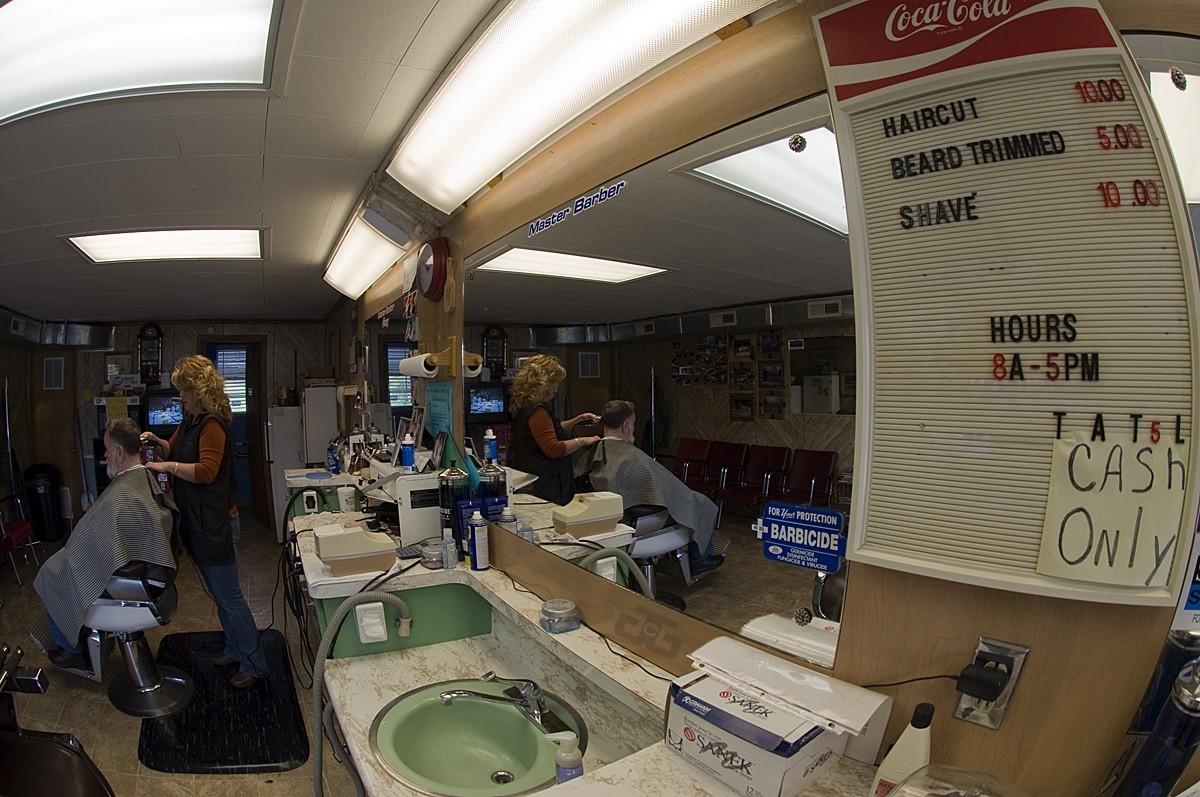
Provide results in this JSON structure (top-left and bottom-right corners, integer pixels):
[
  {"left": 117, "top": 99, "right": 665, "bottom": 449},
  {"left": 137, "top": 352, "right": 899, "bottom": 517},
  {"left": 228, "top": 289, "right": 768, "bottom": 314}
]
[
  {"left": 437, "top": 459, "right": 473, "bottom": 562},
  {"left": 868, "top": 702, "right": 935, "bottom": 797},
  {"left": 476, "top": 457, "right": 508, "bottom": 523},
  {"left": 441, "top": 529, "right": 457, "bottom": 569},
  {"left": 483, "top": 429, "right": 498, "bottom": 467},
  {"left": 519, "top": 518, "right": 533, "bottom": 543},
  {"left": 402, "top": 434, "right": 416, "bottom": 475},
  {"left": 497, "top": 507, "right": 517, "bottom": 536},
  {"left": 467, "top": 510, "right": 489, "bottom": 571}
]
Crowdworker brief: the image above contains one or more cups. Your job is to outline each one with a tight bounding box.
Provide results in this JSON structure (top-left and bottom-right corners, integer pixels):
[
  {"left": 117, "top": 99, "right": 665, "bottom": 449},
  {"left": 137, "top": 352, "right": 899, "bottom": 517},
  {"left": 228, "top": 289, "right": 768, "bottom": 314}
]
[{"left": 337, "top": 487, "right": 355, "bottom": 512}]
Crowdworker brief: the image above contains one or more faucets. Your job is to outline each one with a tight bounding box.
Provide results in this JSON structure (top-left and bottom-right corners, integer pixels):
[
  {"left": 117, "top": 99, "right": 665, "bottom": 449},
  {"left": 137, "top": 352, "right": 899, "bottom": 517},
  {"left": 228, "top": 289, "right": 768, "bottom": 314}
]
[{"left": 435, "top": 669, "right": 549, "bottom": 718}]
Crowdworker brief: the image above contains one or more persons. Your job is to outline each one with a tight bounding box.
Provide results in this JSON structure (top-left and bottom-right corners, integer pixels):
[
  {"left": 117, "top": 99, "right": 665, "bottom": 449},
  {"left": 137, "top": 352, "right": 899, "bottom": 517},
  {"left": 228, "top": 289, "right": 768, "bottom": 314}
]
[
  {"left": 500, "top": 354, "right": 599, "bottom": 510},
  {"left": 42, "top": 418, "right": 173, "bottom": 670},
  {"left": 578, "top": 400, "right": 726, "bottom": 577},
  {"left": 144, "top": 352, "right": 272, "bottom": 685}
]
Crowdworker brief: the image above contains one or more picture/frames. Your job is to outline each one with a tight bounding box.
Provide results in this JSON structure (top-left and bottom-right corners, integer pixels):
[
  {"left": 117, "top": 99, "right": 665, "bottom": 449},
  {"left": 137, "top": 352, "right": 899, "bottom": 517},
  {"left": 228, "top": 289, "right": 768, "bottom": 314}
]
[
  {"left": 397, "top": 417, "right": 411, "bottom": 438},
  {"left": 409, "top": 406, "right": 426, "bottom": 449}
]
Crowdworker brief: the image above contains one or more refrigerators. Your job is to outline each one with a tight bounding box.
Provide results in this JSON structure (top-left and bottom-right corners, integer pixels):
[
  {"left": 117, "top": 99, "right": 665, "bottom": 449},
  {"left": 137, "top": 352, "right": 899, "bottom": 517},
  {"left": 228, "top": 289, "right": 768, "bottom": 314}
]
[{"left": 264, "top": 406, "right": 305, "bottom": 547}]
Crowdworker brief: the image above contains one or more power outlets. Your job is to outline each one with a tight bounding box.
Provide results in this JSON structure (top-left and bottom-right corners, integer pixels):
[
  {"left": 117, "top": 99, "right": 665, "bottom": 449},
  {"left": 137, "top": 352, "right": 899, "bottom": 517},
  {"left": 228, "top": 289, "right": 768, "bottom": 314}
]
[{"left": 952, "top": 636, "right": 1032, "bottom": 730}]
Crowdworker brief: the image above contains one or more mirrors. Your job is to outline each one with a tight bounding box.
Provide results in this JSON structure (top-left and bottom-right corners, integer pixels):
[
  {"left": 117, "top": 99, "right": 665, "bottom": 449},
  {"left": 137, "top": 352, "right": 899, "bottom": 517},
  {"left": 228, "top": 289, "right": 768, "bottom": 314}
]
[
  {"left": 462, "top": 127, "right": 856, "bottom": 671},
  {"left": 364, "top": 292, "right": 415, "bottom": 436}
]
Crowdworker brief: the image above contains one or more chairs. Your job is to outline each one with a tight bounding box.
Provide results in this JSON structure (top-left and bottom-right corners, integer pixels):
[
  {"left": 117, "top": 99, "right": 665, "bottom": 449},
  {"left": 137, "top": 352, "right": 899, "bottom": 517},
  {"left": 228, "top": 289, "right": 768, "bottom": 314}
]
[
  {"left": 0, "top": 496, "right": 193, "bottom": 797},
  {"left": 624, "top": 508, "right": 689, "bottom": 612},
  {"left": 653, "top": 438, "right": 837, "bottom": 528}
]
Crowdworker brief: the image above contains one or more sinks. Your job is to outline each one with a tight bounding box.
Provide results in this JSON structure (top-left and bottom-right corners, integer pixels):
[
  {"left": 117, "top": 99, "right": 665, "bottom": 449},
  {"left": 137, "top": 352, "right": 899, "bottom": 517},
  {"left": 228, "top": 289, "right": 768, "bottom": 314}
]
[{"left": 369, "top": 678, "right": 590, "bottom": 795}]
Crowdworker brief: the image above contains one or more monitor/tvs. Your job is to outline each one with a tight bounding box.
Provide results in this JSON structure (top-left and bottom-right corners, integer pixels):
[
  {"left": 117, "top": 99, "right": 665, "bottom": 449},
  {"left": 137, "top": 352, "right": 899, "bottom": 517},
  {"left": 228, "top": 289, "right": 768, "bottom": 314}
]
[
  {"left": 462, "top": 384, "right": 509, "bottom": 423},
  {"left": 144, "top": 391, "right": 185, "bottom": 431}
]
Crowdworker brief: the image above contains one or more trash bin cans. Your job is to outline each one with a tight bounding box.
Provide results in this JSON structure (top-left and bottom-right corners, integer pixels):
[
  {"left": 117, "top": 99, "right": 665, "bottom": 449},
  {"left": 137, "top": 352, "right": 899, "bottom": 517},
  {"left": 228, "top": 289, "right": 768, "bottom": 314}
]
[{"left": 23, "top": 463, "right": 64, "bottom": 545}]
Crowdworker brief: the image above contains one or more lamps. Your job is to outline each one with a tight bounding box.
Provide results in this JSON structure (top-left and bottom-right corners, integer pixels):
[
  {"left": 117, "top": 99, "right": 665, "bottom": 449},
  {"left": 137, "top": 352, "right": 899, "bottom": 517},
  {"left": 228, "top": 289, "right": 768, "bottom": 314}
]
[
  {"left": 376, "top": 0, "right": 812, "bottom": 231},
  {"left": 320, "top": 170, "right": 435, "bottom": 301}
]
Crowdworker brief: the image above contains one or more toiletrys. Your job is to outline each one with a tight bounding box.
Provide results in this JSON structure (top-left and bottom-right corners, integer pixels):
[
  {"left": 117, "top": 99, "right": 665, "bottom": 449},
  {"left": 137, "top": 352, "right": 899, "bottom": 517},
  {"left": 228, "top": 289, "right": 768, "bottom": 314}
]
[
  {"left": 545, "top": 728, "right": 584, "bottom": 785},
  {"left": 485, "top": 496, "right": 507, "bottom": 523},
  {"left": 458, "top": 499, "right": 482, "bottom": 566},
  {"left": 869, "top": 702, "right": 936, "bottom": 796}
]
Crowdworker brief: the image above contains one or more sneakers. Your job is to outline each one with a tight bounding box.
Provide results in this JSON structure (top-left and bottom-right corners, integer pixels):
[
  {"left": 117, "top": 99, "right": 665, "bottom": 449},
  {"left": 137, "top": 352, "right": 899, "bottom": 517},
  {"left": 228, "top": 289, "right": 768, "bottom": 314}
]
[
  {"left": 230, "top": 671, "right": 257, "bottom": 688},
  {"left": 690, "top": 555, "right": 725, "bottom": 581},
  {"left": 213, "top": 650, "right": 235, "bottom": 666},
  {"left": 48, "top": 647, "right": 91, "bottom": 670}
]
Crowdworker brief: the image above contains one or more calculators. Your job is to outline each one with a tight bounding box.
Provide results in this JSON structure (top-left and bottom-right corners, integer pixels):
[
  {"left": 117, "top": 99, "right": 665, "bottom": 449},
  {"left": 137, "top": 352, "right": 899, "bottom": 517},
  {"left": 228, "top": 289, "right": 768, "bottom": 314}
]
[{"left": 396, "top": 540, "right": 433, "bottom": 559}]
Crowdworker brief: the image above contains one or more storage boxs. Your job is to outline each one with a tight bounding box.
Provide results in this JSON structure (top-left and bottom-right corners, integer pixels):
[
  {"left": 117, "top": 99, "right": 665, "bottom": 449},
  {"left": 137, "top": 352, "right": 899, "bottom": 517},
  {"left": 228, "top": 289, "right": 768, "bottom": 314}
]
[{"left": 664, "top": 670, "right": 850, "bottom": 797}]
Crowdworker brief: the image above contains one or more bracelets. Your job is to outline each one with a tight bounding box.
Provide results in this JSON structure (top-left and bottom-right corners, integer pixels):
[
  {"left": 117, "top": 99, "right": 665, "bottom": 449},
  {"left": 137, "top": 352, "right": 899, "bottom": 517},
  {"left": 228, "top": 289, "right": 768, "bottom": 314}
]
[
  {"left": 573, "top": 436, "right": 582, "bottom": 448},
  {"left": 171, "top": 461, "right": 180, "bottom": 478}
]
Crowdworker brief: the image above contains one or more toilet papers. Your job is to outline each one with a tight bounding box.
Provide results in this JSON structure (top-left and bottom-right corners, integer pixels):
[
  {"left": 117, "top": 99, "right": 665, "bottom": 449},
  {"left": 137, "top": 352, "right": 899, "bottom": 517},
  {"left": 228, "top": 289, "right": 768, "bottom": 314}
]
[{"left": 399, "top": 352, "right": 440, "bottom": 379}]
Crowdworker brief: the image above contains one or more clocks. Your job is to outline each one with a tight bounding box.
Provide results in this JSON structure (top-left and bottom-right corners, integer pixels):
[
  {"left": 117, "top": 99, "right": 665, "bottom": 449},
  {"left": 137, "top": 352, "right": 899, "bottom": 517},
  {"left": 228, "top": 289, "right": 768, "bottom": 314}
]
[{"left": 417, "top": 237, "right": 448, "bottom": 304}]
